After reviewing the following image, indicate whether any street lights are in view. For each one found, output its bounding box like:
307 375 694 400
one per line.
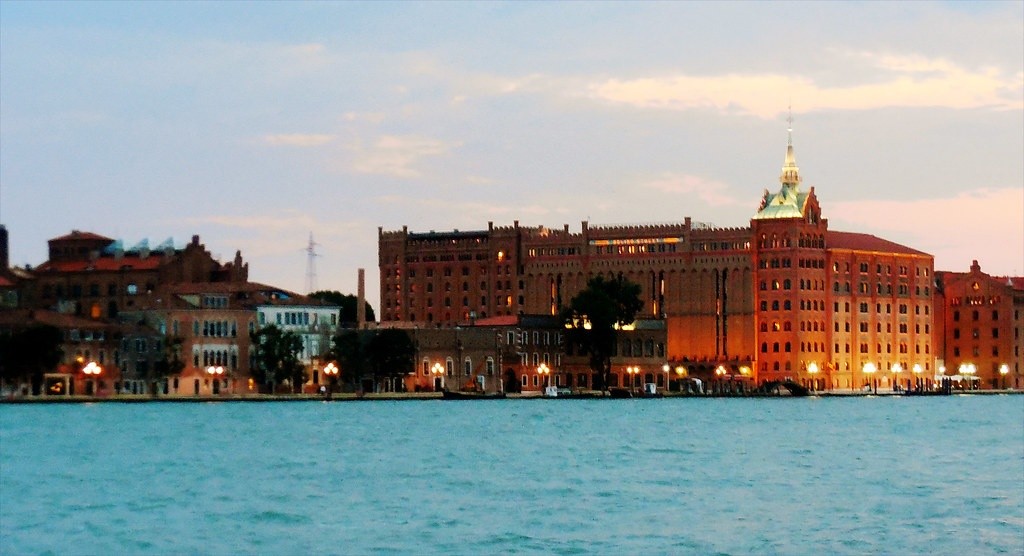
913 365 921 390
808 363 818 391
716 366 726 393
431 363 444 391
83 361 102 396
207 366 224 398
537 364 550 396
862 362 876 392
627 366 639 393
662 364 670 390
960 364 976 390
1000 365 1009 390
892 364 902 390
324 362 339 394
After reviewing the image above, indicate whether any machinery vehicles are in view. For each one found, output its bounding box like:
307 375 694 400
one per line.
462 355 488 392
